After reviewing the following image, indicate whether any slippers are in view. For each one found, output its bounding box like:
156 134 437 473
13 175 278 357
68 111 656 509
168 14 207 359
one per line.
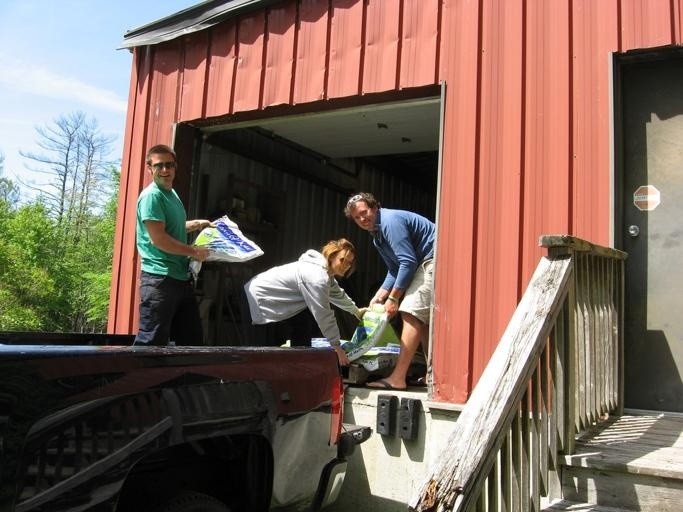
365 379 408 391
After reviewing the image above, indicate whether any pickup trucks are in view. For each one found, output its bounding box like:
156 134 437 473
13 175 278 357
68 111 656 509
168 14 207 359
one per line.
0 332 372 511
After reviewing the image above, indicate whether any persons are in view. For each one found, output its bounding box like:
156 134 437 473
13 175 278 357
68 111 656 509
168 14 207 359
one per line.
240 237 370 368
344 191 435 390
132 143 219 346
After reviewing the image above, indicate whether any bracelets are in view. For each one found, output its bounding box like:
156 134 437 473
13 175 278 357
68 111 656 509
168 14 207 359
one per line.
389 295 397 303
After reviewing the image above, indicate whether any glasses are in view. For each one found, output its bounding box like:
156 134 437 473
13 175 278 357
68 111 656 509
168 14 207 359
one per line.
152 161 176 169
